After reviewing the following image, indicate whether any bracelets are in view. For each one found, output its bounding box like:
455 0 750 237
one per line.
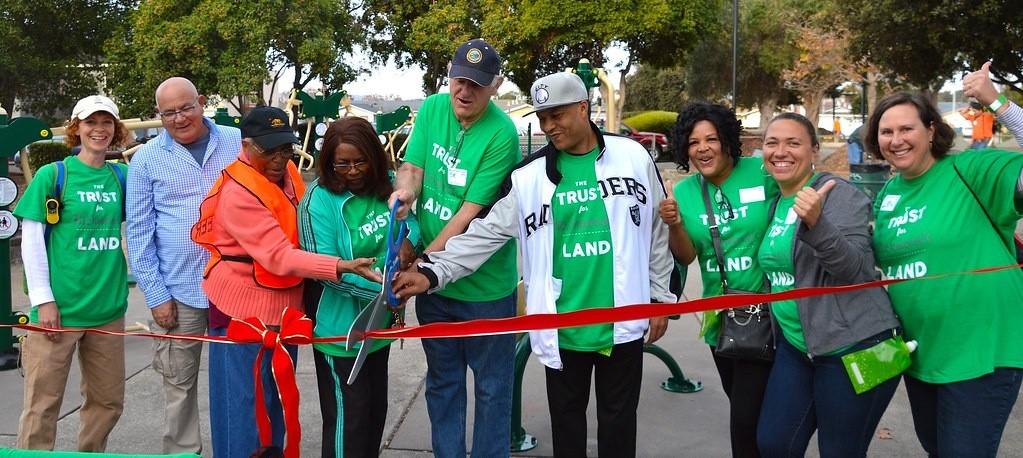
989 93 1008 112
419 251 434 265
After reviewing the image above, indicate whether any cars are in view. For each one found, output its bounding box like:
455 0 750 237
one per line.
532 118 670 163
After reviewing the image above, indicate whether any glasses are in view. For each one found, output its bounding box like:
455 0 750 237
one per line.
333 161 370 174
251 144 294 158
158 97 198 122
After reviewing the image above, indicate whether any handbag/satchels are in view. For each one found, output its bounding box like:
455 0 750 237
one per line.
715 287 775 364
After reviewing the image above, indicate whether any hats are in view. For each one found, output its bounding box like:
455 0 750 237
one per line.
71 95 120 123
240 106 300 150
520 72 588 118
449 38 500 87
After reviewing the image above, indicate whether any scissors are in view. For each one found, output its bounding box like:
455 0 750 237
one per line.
346 198 406 387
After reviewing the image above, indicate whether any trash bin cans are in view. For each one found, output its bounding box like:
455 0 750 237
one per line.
850 163 891 207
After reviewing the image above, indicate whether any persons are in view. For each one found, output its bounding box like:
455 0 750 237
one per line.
754 112 905 458
10 95 136 454
393 74 680 458
848 123 865 163
834 116 841 143
386 41 519 458
659 100 780 458
190 98 384 458
298 115 420 458
865 60 1023 458
124 76 244 458
961 101 996 150
